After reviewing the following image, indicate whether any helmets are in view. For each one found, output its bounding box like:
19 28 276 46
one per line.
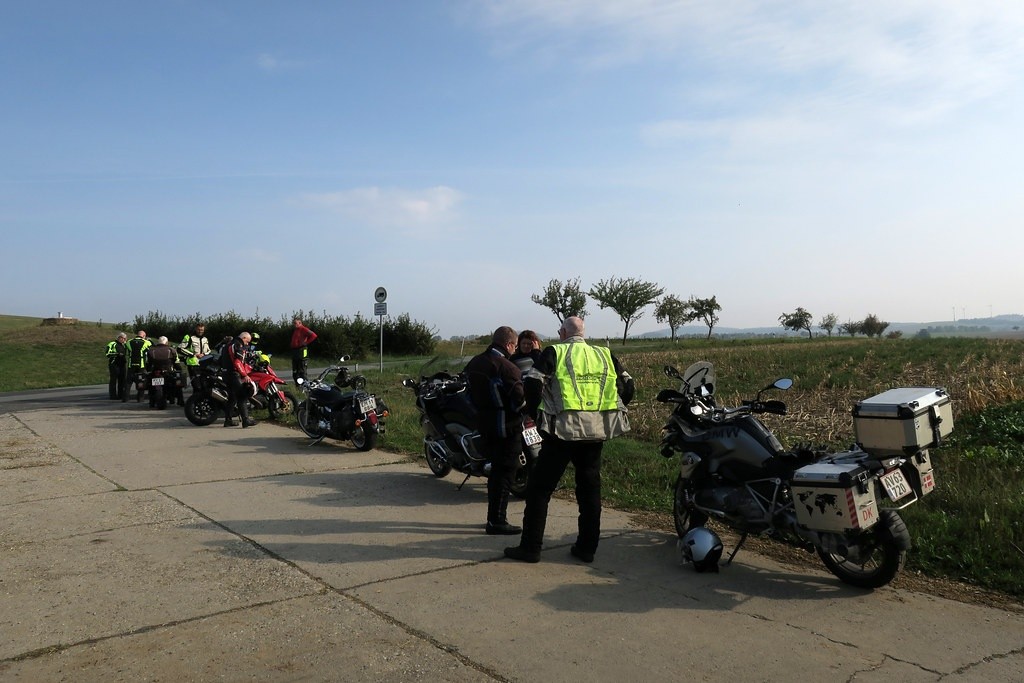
334 369 350 389
250 333 260 344
681 528 722 567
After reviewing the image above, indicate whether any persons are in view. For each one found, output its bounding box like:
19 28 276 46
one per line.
510 330 544 412
122 331 152 403
178 323 212 383
220 332 259 428
291 319 317 387
466 327 525 534
105 333 126 400
504 317 636 562
148 336 184 406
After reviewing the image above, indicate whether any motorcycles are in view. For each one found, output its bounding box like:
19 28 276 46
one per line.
401 355 546 499
296 354 390 452
656 360 955 590
134 354 183 410
183 352 299 426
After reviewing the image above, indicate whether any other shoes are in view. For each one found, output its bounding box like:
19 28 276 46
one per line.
571 545 595 564
176 399 186 406
485 517 525 535
242 419 259 428
138 399 146 403
503 545 539 563
224 420 239 427
